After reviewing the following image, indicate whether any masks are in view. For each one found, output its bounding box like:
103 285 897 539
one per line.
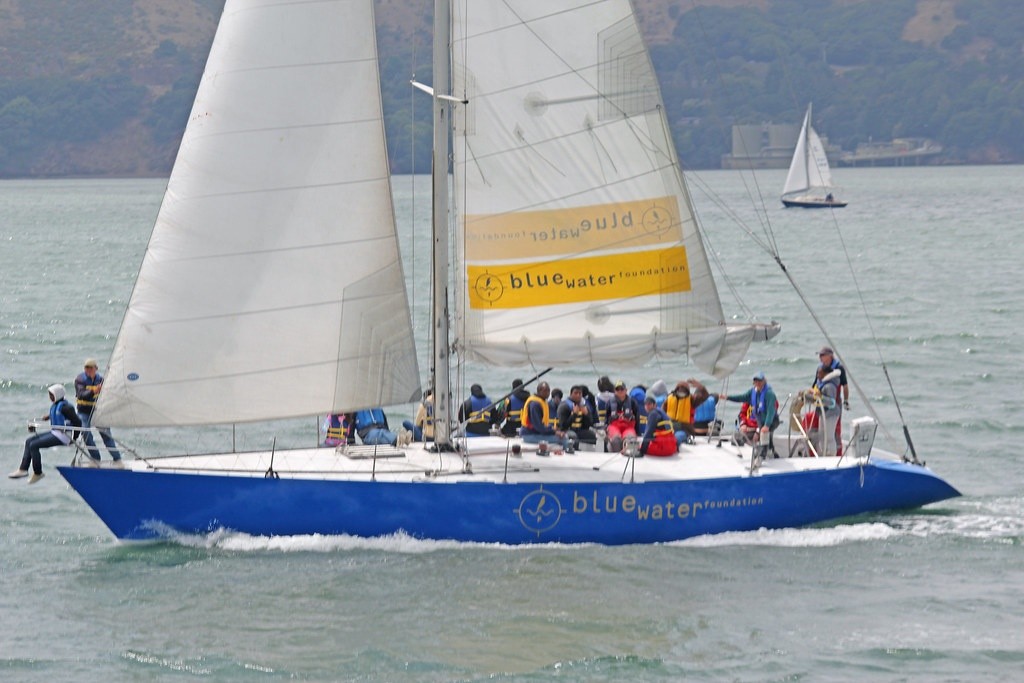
677 390 688 398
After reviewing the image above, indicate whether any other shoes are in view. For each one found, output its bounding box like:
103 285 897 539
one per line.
610 435 622 452
89 459 101 468
8 469 29 478
112 459 125 470
623 432 636 452
395 427 413 448
29 472 45 484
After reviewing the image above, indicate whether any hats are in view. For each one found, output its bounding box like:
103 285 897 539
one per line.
752 371 765 380
815 346 834 356
644 393 656 403
84 359 97 368
614 380 626 390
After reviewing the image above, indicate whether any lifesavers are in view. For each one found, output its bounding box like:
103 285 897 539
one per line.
790 396 806 431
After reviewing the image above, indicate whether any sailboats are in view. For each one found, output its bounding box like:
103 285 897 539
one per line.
55 0 963 545
781 102 849 207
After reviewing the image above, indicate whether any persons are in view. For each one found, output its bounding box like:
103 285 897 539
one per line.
548 389 563 428
809 347 849 455
519 381 570 450
495 379 530 437
403 389 434 442
579 386 600 424
7 383 82 483
355 407 413 449
595 376 724 457
557 384 608 452
320 412 356 448
458 384 497 436
75 357 124 469
719 371 780 459
